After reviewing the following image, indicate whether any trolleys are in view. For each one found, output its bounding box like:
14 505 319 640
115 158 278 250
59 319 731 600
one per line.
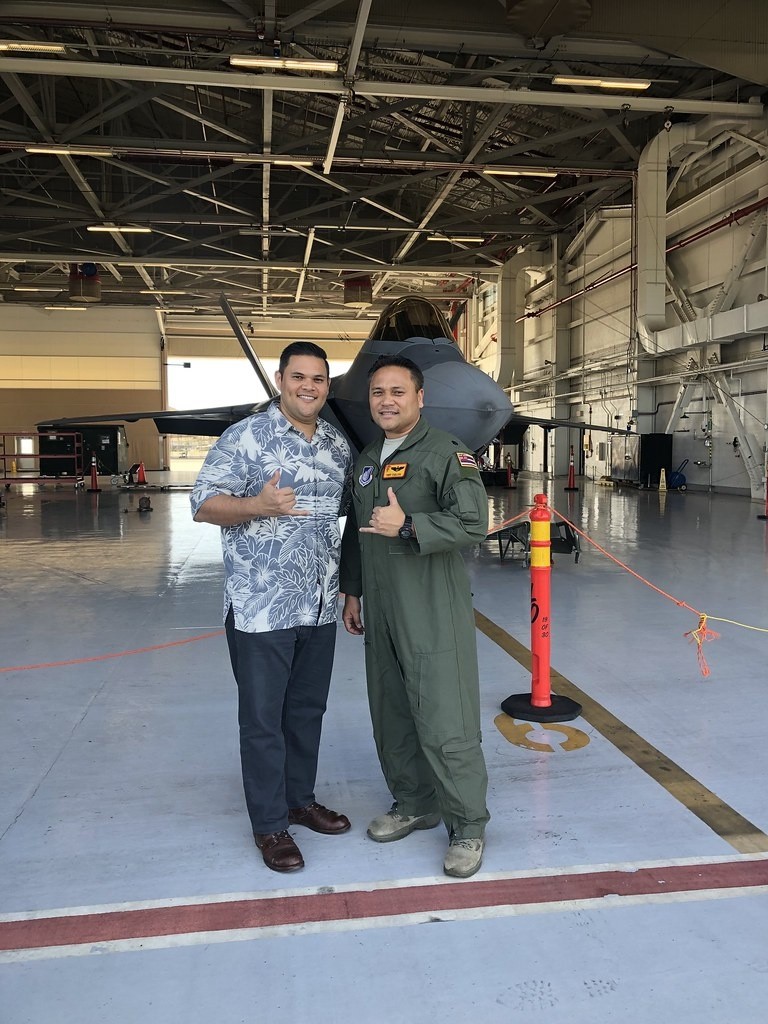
669 458 690 492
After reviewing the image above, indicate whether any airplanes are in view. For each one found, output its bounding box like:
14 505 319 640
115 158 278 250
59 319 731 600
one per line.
34 293 644 466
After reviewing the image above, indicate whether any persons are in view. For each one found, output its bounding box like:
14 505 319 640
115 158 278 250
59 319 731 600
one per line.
504 452 512 464
188 342 354 873
339 355 489 878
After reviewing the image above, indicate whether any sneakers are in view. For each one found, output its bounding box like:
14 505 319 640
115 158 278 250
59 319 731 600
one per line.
366 810 442 843
444 838 485 878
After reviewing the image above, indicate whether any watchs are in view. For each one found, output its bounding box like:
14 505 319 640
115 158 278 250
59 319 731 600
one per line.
398 516 413 540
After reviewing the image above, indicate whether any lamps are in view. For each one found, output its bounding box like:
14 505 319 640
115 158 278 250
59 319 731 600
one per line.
551 73 651 89
87 222 151 232
239 225 300 236
25 144 114 157
229 55 338 70
233 157 313 166
138 289 186 294
13 285 63 292
427 232 484 242
483 166 557 177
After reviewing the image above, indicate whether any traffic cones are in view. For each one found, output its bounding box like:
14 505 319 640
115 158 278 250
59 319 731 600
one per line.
658 468 668 492
500 453 519 490
563 446 578 491
86 450 104 494
11 461 18 473
135 461 148 485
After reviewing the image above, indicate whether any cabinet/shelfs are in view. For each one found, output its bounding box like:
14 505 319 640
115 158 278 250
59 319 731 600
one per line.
610 432 673 482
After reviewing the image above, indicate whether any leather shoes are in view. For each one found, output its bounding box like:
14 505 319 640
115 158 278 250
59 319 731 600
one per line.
288 801 350 835
254 830 305 872
